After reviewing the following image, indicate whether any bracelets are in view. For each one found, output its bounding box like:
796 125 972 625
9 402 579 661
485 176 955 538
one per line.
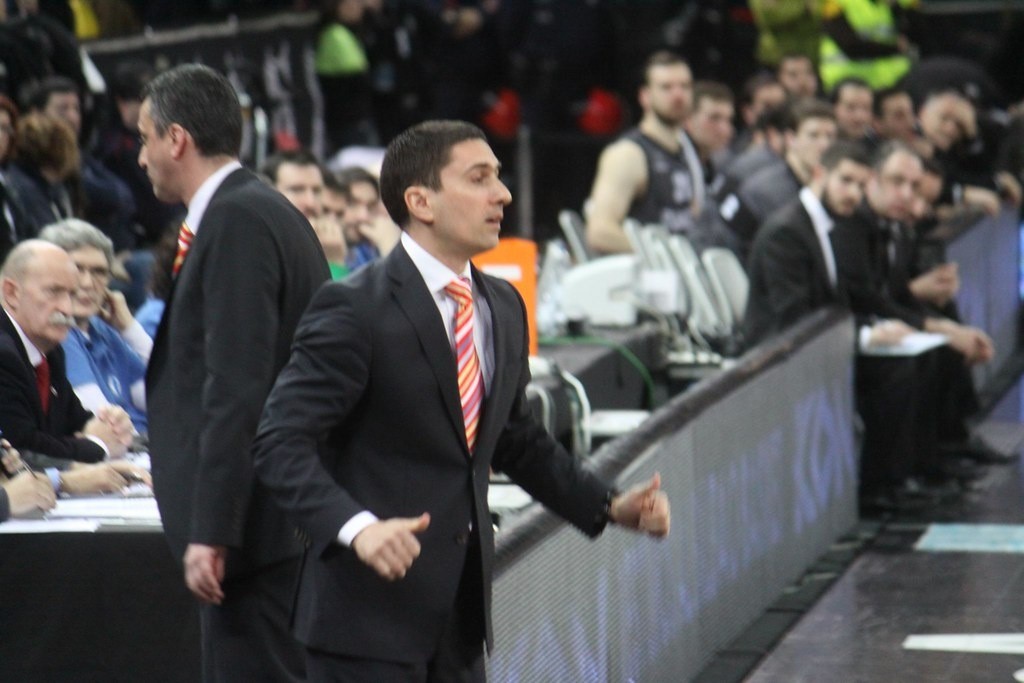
44 467 69 498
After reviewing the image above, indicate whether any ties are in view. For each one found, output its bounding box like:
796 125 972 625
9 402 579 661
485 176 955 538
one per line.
442 277 486 458
173 220 193 281
32 355 51 415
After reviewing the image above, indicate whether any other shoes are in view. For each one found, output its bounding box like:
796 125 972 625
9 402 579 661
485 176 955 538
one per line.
859 432 1005 523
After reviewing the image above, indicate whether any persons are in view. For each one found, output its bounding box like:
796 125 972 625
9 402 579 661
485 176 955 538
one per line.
248 121 668 683
135 61 333 683
0 0 393 526
314 0 1024 515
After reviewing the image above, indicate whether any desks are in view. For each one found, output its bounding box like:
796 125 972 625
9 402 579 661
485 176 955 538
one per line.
0 320 667 683
